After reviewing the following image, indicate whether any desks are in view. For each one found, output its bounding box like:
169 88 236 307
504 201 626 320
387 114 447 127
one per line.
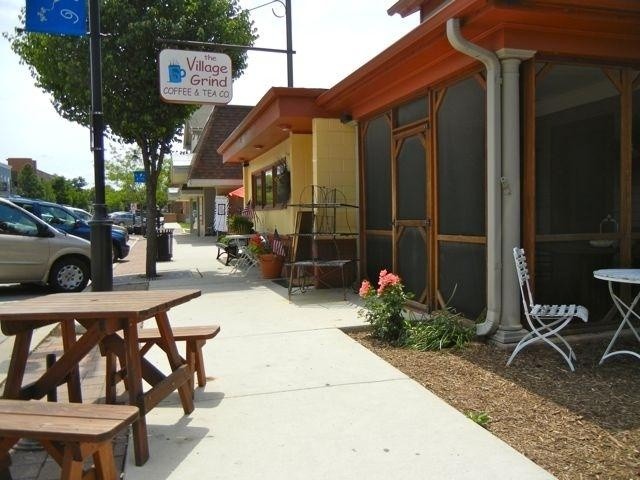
593 263 640 369
0 292 199 468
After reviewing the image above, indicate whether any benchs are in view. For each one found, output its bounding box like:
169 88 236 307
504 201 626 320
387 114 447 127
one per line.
216 239 239 267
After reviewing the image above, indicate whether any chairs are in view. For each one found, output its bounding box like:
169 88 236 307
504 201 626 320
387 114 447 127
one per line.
504 245 589 372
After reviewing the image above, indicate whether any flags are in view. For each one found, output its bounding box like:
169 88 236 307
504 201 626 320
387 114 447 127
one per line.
242 201 256 219
272 229 289 257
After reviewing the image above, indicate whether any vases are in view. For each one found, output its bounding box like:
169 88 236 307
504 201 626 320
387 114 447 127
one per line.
259 253 283 279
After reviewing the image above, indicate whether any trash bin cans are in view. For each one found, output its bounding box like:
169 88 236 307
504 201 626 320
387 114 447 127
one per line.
157 229 173 262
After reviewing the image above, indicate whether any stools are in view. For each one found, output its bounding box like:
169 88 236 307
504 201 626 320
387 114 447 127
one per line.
0 396 140 475
114 326 223 399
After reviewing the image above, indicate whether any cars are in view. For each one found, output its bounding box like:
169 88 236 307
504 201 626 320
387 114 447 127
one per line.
0 198 91 292
10 199 146 258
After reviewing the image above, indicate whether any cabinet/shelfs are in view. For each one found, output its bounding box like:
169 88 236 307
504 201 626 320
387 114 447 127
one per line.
281 183 363 302
